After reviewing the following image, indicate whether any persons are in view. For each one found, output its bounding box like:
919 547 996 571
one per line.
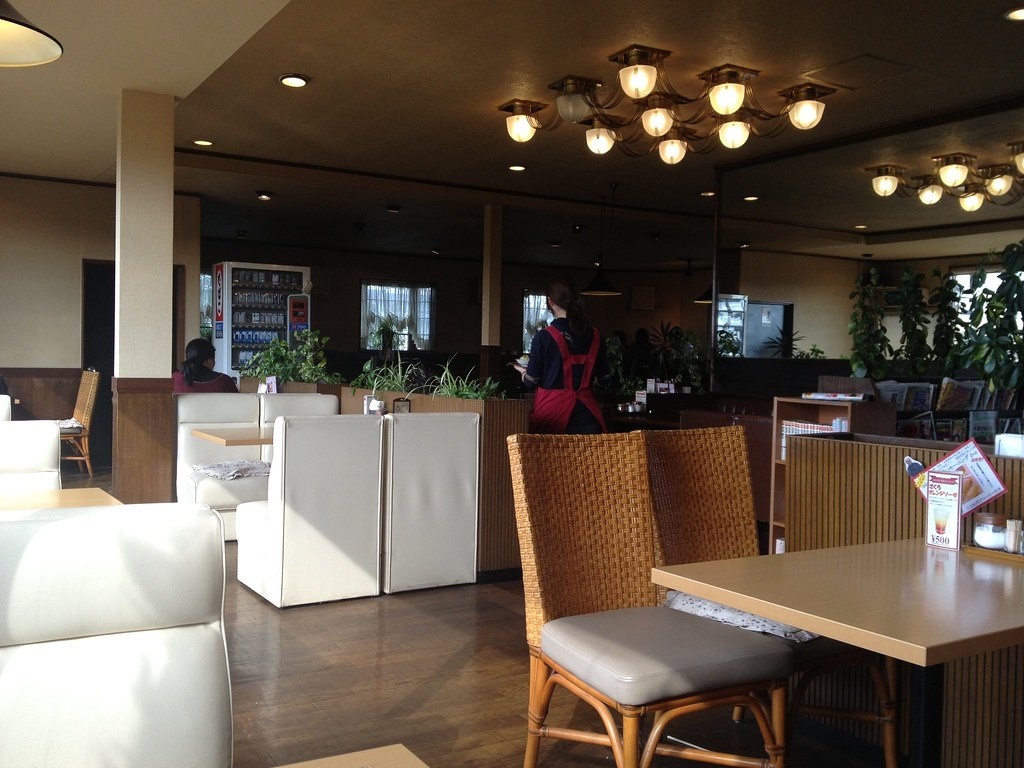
667 326 698 357
514 278 607 435
614 328 656 363
173 338 238 392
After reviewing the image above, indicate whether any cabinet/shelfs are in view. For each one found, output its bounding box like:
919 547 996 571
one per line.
767 394 897 557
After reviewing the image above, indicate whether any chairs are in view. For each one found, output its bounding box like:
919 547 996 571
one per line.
506 432 795 768
642 426 901 768
58 370 100 478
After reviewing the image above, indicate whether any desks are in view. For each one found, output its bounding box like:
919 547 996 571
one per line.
651 536 1024 768
0 487 125 512
191 428 274 446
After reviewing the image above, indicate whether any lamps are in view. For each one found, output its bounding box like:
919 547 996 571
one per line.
580 196 623 296
497 44 836 166
865 140 1024 211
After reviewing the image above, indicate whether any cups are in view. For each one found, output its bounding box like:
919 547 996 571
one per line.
934 507 949 534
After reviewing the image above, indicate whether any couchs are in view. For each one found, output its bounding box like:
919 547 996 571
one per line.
0 502 234 768
0 420 62 491
175 391 480 609
679 407 773 524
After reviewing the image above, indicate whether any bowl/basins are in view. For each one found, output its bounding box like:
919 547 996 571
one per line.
516 359 529 367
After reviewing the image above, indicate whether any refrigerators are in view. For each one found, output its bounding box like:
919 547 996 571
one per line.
212 261 310 394
